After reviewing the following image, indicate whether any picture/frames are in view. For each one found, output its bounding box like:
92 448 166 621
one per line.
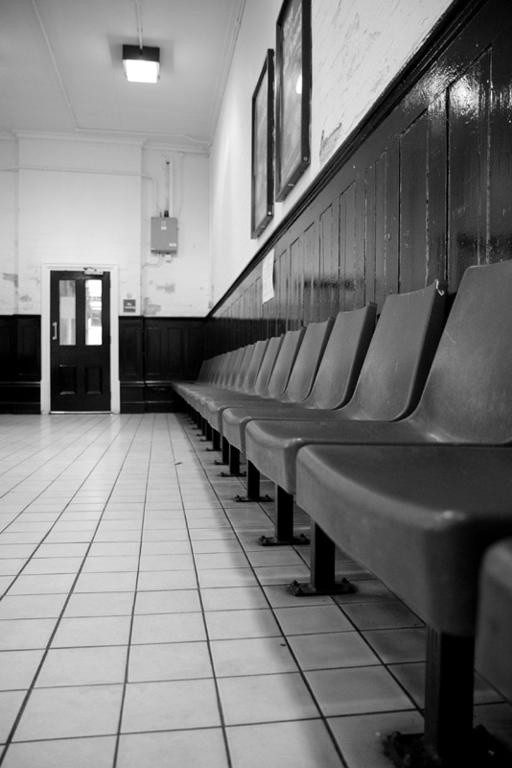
250 0 309 239
